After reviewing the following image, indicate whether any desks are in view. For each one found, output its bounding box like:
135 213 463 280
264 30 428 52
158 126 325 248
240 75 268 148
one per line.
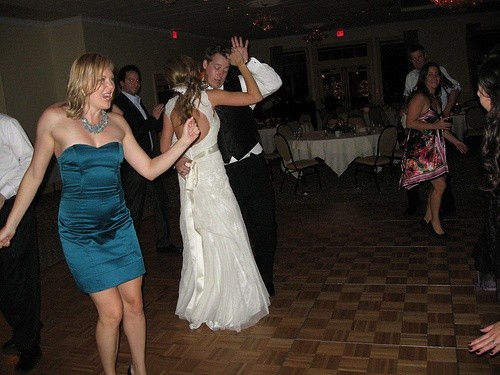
254 119 287 154
452 108 472 142
281 127 400 178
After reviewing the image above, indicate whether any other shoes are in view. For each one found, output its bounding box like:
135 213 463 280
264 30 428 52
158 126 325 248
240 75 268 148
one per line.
404 200 423 217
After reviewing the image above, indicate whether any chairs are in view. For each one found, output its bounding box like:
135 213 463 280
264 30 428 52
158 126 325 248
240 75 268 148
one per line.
464 107 488 145
264 107 402 199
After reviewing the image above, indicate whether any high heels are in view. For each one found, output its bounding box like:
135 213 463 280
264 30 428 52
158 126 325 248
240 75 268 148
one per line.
421 218 429 230
428 221 447 238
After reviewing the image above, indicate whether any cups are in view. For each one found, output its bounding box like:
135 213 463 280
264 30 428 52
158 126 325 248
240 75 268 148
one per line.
353 126 357 135
444 118 453 129
324 130 328 136
368 121 382 135
335 131 342 138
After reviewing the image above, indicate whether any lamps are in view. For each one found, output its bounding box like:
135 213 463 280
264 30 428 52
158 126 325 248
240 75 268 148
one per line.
250 4 284 33
302 16 328 47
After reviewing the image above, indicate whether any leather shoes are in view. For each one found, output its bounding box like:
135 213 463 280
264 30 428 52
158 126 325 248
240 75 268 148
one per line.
2 341 16 351
157 243 182 253
14 349 42 373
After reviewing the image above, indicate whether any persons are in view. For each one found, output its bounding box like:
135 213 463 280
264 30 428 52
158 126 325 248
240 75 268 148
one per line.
115 65 178 254
403 43 462 191
0 113 43 375
470 44 500 304
398 61 469 238
358 97 389 127
0 54 200 375
161 47 270 332
468 321 500 356
202 36 282 292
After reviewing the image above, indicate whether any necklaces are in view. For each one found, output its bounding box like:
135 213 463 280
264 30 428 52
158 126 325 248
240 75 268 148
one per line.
79 110 108 133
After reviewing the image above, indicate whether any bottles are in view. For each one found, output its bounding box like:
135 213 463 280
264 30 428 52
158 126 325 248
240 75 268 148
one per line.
296 127 302 140
